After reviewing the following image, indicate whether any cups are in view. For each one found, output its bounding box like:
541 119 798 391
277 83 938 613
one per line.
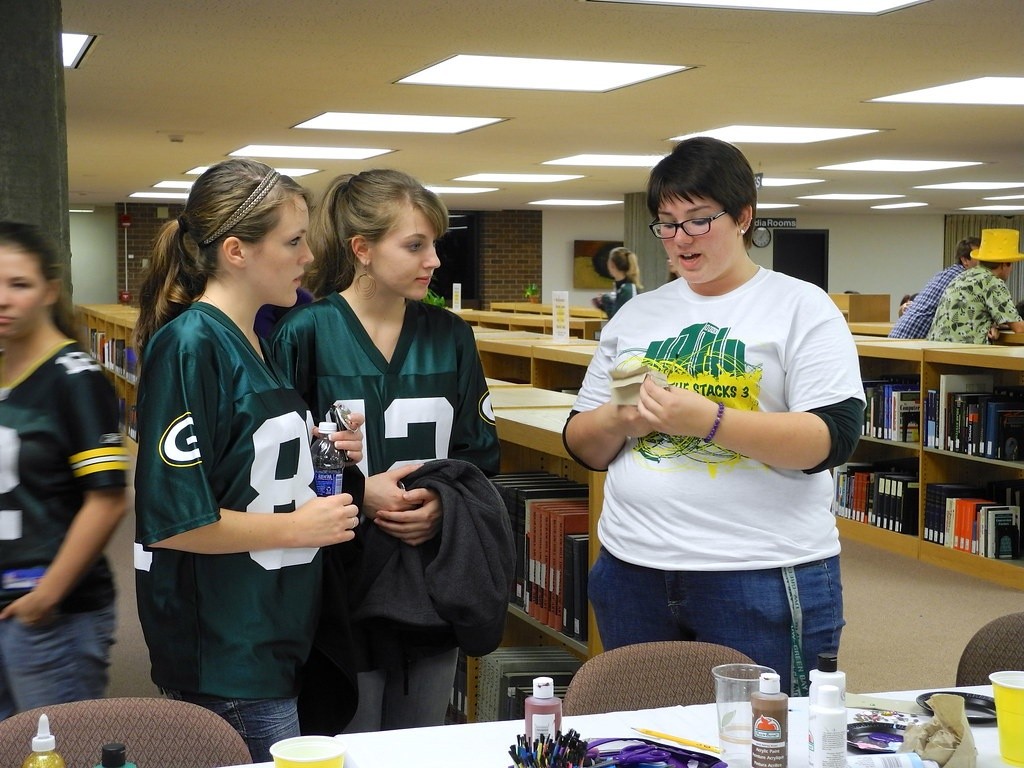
269 735 347 768
988 670 1024 768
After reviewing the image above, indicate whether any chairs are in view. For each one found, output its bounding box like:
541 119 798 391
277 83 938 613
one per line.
956 612 1024 688
561 641 762 716
0 698 254 768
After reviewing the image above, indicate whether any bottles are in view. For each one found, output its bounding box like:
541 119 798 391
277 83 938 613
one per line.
807 653 849 768
94 743 137 768
310 422 347 497
711 663 777 745
749 674 788 767
22 714 67 768
525 677 562 750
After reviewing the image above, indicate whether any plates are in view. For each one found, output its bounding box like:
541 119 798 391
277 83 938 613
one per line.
846 722 907 753
915 691 997 724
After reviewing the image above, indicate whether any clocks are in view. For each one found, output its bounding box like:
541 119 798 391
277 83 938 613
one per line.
752 226 771 248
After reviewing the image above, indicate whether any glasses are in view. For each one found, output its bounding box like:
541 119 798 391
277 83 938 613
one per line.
649 208 728 239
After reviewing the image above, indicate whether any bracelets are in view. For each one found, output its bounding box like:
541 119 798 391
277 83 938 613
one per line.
703 402 725 444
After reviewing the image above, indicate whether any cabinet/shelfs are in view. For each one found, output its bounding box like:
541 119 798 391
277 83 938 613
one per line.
73 294 1024 727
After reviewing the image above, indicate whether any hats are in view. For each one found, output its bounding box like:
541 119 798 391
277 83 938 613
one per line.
970 229 1024 263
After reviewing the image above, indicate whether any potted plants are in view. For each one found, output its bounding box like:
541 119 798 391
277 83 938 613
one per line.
526 284 539 303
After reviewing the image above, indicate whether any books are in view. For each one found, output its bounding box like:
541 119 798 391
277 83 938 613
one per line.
446 645 584 722
487 472 591 642
831 374 1024 559
82 327 141 443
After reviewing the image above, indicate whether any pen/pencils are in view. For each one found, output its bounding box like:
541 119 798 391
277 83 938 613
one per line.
630 726 726 755
510 727 589 768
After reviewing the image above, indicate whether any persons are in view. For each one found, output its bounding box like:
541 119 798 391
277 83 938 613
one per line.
0 220 132 720
562 137 867 697
926 228 1024 345
592 247 641 317
268 169 500 735
130 159 366 764
887 236 981 339
666 257 681 281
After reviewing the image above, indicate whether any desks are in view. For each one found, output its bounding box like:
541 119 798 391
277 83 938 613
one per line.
332 686 1024 768
